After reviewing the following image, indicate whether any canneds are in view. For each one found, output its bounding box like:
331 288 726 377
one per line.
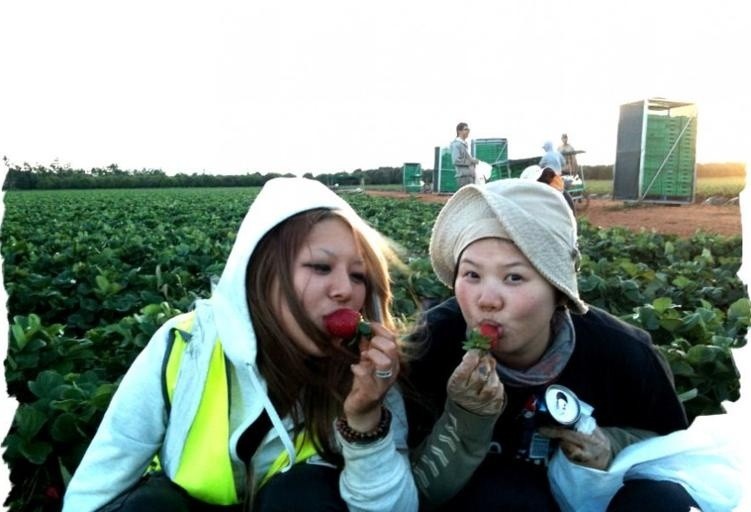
513 383 581 467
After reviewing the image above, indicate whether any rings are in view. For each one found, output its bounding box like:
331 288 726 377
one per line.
374 367 394 378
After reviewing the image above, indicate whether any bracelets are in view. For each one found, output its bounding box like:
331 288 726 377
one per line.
335 403 392 444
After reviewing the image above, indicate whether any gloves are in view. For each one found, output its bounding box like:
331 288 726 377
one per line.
446 348 505 416
540 426 611 469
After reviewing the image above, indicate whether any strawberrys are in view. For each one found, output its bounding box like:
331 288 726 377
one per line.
322 308 364 337
474 322 499 350
44 484 62 508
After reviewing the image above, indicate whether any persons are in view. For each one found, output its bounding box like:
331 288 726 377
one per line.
396 175 700 512
538 167 556 185
539 140 566 178
59 175 420 512
449 122 493 187
557 132 578 173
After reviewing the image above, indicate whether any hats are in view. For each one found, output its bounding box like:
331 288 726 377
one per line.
427 178 588 316
